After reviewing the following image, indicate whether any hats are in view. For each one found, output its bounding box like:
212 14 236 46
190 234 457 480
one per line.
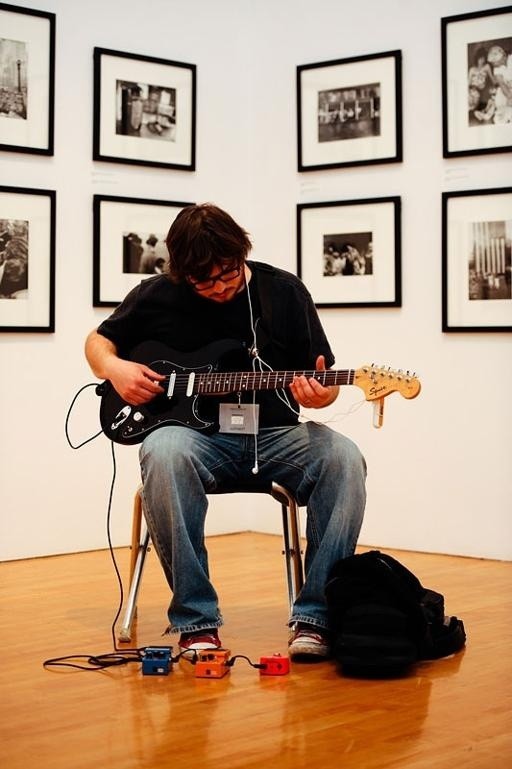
146 234 157 244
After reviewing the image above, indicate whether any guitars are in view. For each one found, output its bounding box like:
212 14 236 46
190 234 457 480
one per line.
99 361 421 444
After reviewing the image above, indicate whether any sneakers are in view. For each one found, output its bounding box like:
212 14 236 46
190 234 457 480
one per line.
288 620 330 659
178 628 222 661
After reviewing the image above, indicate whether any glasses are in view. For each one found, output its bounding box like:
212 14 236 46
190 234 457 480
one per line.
187 259 241 291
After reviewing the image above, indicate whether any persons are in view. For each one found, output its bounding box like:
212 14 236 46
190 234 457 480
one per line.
121 232 169 275
85 203 369 663
321 240 372 277
468 45 512 126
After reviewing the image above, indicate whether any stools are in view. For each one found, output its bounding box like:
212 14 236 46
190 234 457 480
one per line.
120 473 304 649
